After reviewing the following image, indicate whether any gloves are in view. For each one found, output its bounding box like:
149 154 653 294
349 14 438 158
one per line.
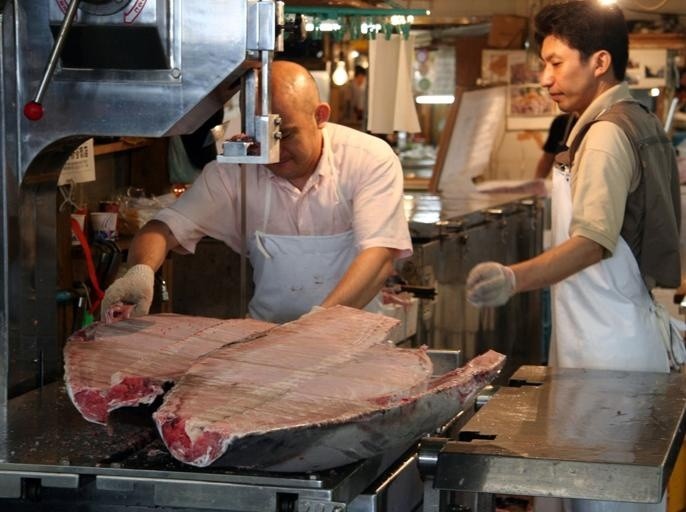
462 258 518 313
99 261 156 326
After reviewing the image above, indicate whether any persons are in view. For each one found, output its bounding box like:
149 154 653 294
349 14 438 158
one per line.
465 0 681 512
101 59 415 328
340 65 366 121
536 113 578 179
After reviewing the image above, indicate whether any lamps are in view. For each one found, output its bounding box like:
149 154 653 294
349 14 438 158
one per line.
331 31 349 86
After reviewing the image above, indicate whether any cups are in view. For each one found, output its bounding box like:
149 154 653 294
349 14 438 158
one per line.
90 210 118 243
70 213 86 246
